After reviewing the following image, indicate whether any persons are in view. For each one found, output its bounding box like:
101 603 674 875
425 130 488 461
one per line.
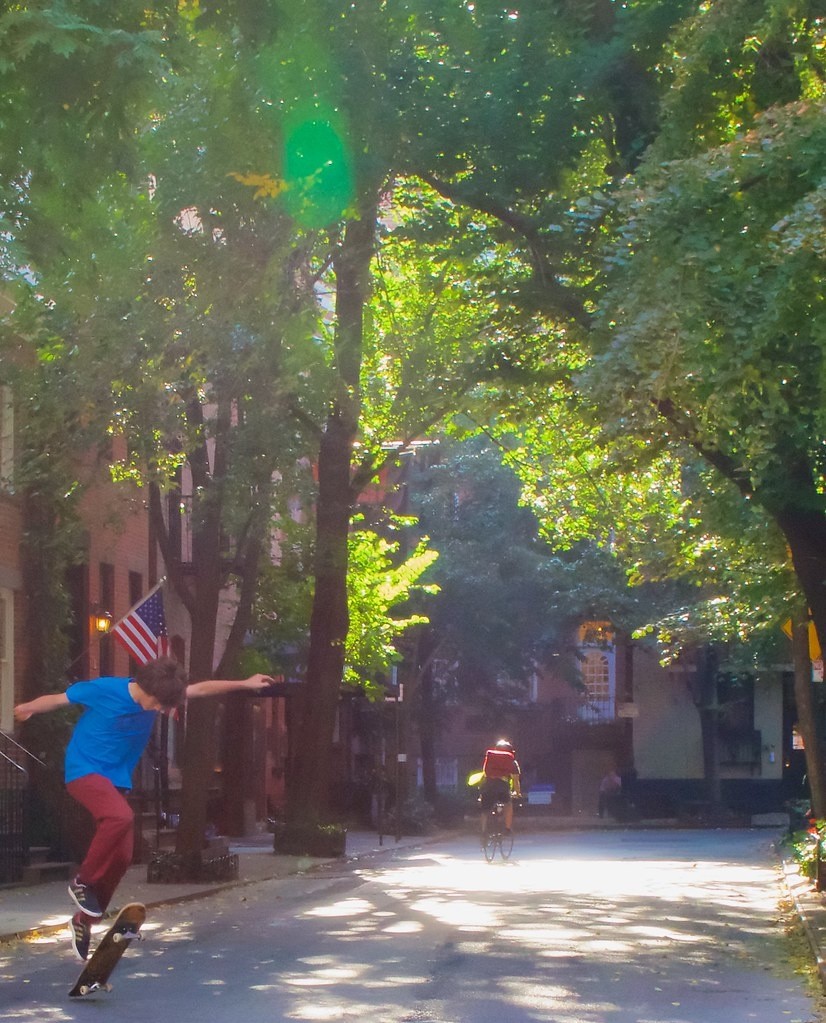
10 674 273 962
597 768 621 817
480 739 521 849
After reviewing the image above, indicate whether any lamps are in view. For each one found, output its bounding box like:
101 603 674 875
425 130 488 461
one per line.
91 602 113 631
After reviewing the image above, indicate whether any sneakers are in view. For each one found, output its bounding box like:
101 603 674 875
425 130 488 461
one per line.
68 876 103 917
69 913 91 962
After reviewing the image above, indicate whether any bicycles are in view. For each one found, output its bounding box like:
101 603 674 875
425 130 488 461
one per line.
477 787 521 863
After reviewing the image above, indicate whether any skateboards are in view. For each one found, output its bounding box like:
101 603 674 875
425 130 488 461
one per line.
66 901 148 998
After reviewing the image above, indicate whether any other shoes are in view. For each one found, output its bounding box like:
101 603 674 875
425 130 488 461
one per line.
504 830 513 838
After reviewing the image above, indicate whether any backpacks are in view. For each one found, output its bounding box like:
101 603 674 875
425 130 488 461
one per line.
484 749 515 779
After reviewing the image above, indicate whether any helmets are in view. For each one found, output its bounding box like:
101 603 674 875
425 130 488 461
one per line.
496 741 512 752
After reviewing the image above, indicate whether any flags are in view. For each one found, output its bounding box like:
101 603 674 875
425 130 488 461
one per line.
111 587 179 721
